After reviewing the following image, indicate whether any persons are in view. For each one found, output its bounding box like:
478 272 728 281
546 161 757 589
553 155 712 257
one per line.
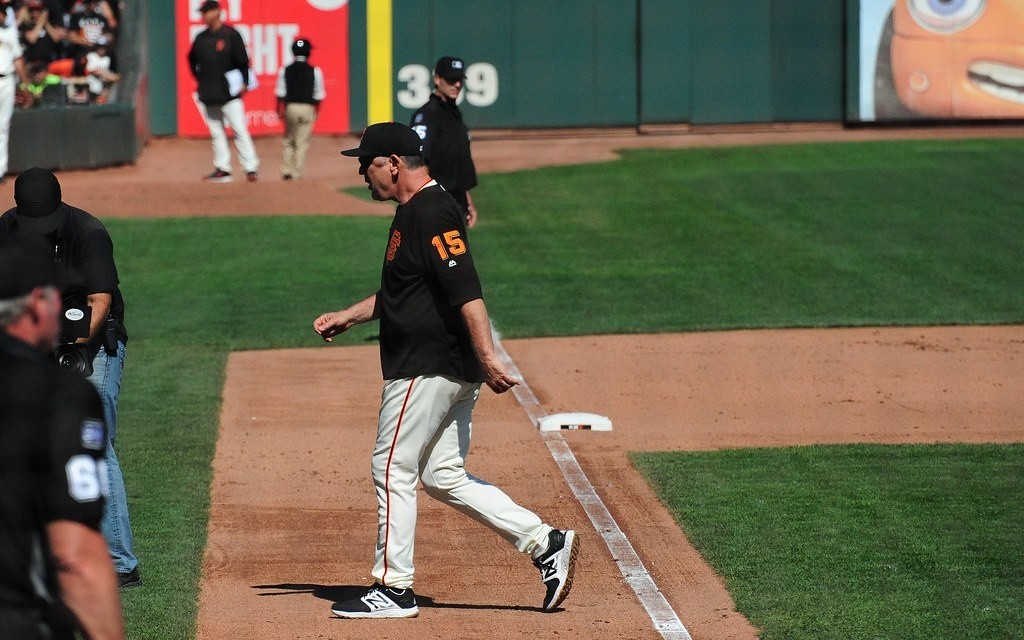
189 0 260 183
0 169 145 640
273 39 326 181
409 56 478 230
0 0 121 184
313 120 583 620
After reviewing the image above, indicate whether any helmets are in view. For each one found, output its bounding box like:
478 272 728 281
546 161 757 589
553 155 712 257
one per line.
292 40 310 57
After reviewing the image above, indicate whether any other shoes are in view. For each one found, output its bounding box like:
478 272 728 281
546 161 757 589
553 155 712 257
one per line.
283 174 292 180
116 566 142 589
201 167 233 183
247 172 257 182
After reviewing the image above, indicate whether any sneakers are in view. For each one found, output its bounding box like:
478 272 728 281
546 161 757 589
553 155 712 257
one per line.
331 582 419 619
532 529 580 612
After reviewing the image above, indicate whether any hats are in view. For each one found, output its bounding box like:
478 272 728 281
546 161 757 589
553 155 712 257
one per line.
198 0 219 14
436 56 467 83
0 215 84 290
340 120 423 158
14 167 62 234
